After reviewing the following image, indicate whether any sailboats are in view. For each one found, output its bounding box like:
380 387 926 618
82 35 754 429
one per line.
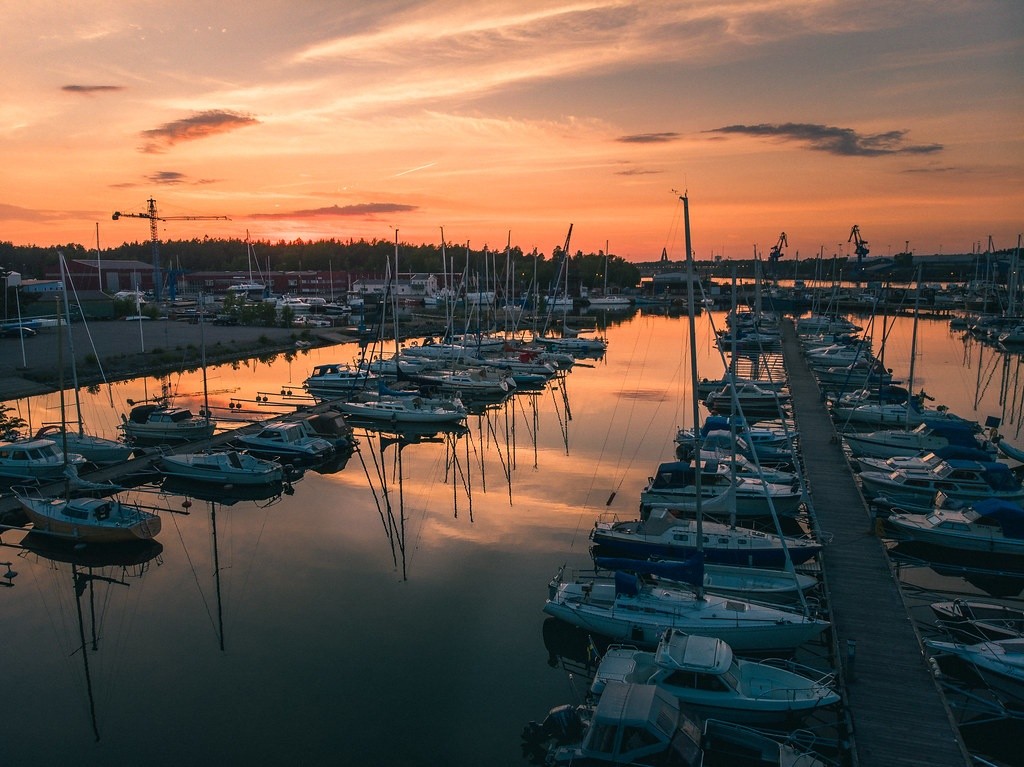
0 190 1024 766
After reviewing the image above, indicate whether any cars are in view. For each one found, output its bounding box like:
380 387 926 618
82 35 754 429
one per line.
0 326 37 339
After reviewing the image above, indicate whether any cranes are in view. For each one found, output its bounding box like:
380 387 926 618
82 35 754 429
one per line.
110 197 233 303
767 231 788 288
847 225 870 287
